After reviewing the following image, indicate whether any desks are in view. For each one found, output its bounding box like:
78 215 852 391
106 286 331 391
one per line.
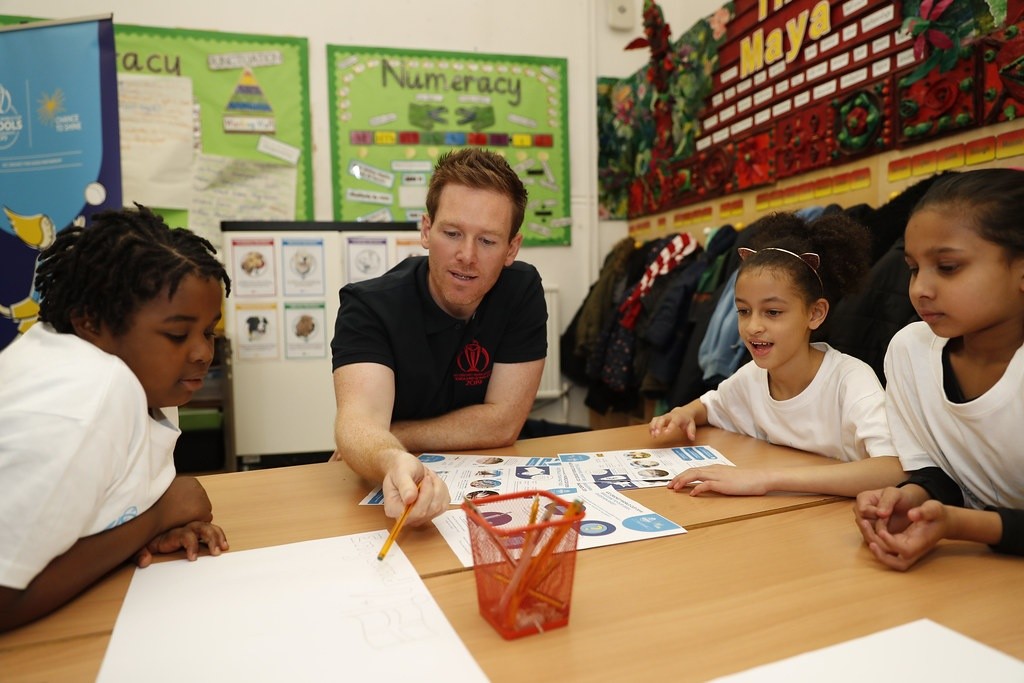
177 328 238 472
0 423 1024 683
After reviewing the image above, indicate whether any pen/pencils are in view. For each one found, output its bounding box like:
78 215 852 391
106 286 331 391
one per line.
375 475 430 562
462 491 587 631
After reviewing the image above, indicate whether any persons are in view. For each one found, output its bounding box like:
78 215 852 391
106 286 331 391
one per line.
0 200 232 630
649 212 910 497
853 168 1023 569
330 149 549 527
